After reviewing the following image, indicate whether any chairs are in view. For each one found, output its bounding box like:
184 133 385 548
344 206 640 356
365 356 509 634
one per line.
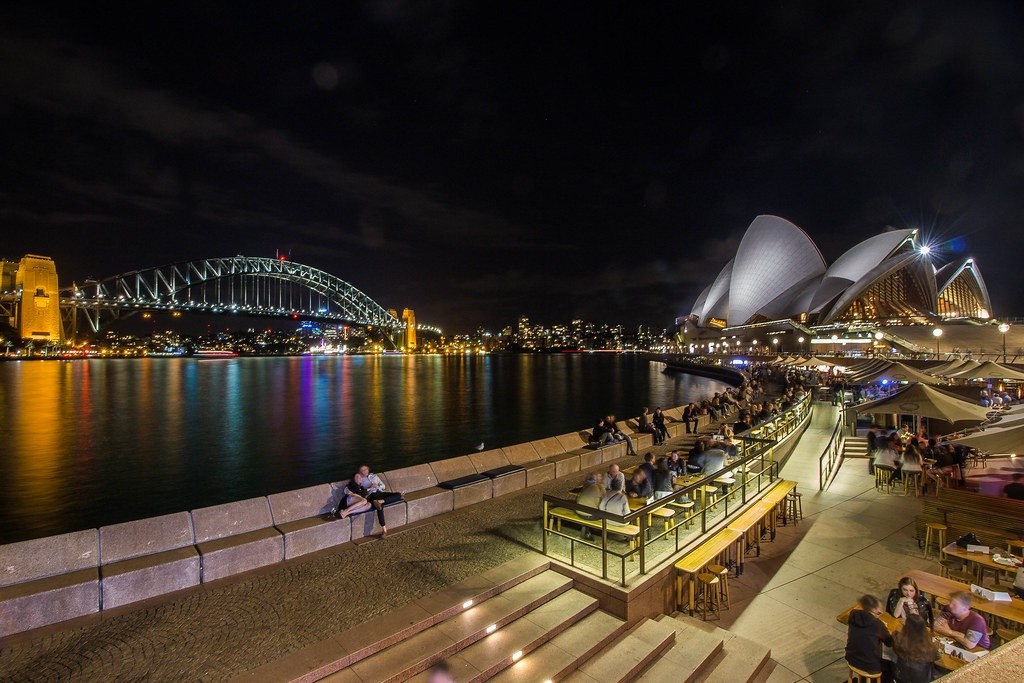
965 447 978 467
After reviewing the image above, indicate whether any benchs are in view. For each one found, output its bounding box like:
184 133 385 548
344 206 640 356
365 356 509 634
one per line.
674 528 744 618
762 480 798 537
0 394 811 639
727 499 777 556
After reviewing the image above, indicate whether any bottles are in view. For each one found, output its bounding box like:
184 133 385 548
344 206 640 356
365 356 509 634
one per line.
907 596 916 614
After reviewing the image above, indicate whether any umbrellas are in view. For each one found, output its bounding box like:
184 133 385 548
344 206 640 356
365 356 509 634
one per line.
767 356 1024 455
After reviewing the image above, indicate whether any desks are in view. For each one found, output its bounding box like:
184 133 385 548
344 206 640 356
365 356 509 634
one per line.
834 602 988 670
864 437 909 459
623 466 703 516
570 486 648 550
897 570 1024 630
942 542 1023 583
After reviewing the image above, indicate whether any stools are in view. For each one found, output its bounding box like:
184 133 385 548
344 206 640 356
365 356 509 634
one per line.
922 522 948 561
845 661 883 683
784 496 798 526
874 466 959 496
788 491 802 520
697 573 722 621
707 565 729 610
927 559 1022 645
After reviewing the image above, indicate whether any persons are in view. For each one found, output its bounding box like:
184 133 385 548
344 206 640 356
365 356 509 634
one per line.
336 473 373 518
844 380 1024 683
344 464 402 535
575 358 848 541
710 347 1024 358
